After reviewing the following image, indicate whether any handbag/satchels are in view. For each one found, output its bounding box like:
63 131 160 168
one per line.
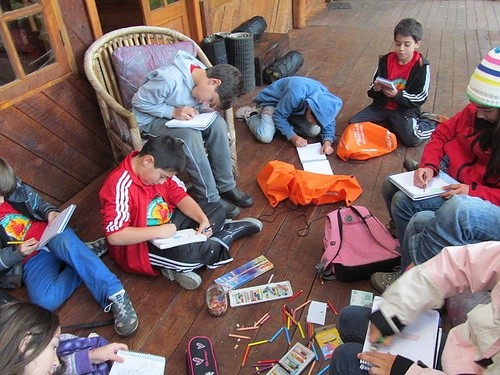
337 121 398 163
256 160 363 207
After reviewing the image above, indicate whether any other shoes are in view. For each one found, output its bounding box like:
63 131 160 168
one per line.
214 186 254 217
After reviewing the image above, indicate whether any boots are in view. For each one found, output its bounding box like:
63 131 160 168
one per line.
211 217 263 252
160 266 204 290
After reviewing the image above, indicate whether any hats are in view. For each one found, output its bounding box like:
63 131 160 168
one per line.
466 45 500 108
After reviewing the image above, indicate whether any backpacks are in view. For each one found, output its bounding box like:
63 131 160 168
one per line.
317 205 404 282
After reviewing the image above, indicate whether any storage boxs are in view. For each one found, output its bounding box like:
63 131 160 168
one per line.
252 31 291 86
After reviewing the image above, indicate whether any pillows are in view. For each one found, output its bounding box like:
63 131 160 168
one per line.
112 41 198 110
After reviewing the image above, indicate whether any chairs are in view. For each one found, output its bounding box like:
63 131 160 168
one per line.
83 25 239 196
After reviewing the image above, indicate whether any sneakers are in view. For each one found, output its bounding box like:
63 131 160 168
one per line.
235 104 255 122
108 288 139 337
421 110 450 124
84 236 108 257
369 268 402 294
402 158 421 171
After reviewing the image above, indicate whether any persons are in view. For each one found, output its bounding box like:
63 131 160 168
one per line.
327 239 500 375
98 134 264 290
129 49 255 218
379 45 500 262
235 75 344 156
0 156 139 336
369 193 500 325
8 14 55 83
348 16 452 148
1 300 129 375
